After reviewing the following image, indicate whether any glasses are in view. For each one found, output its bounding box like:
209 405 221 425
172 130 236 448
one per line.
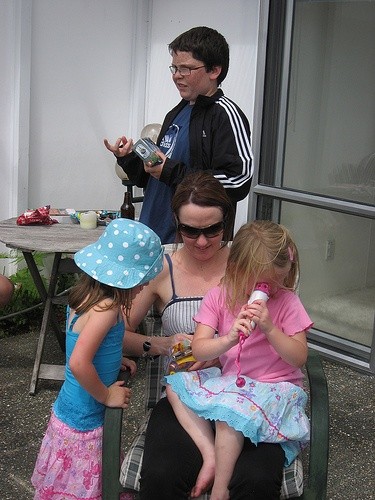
178 220 224 238
169 62 215 76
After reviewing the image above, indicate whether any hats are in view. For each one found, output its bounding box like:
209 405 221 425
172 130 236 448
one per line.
74 218 166 288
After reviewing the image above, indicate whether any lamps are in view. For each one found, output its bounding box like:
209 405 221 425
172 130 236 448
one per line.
115 123 163 202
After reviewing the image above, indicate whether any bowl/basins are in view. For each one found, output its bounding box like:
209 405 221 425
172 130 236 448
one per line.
50 215 78 225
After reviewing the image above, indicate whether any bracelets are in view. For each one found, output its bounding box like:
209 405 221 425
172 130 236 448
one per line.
143 336 154 356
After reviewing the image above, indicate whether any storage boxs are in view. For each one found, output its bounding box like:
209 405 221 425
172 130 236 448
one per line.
131 137 162 166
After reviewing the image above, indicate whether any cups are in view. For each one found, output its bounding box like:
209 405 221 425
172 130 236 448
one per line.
80 214 100 230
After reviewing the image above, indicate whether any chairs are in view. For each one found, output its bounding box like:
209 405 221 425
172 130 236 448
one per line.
100 242 330 500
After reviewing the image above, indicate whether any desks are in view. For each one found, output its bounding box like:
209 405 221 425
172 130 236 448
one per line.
0 208 123 396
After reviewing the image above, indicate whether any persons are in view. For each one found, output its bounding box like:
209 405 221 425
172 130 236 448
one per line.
103 26 254 241
162 218 314 500
29 219 165 500
122 170 286 500
